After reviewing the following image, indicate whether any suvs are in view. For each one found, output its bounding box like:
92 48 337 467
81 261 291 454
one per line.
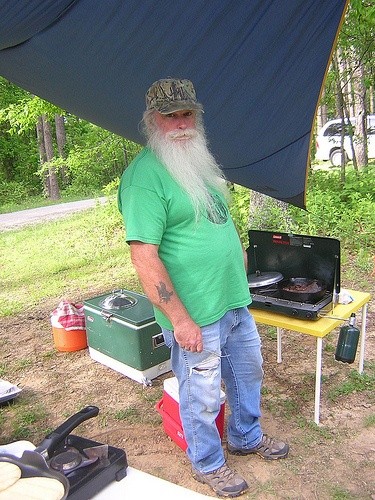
314 115 375 167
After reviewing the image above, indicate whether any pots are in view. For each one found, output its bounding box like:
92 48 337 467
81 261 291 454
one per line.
258 278 327 304
0 406 99 500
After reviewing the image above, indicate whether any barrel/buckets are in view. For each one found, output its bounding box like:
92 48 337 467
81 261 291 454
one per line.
50 303 87 352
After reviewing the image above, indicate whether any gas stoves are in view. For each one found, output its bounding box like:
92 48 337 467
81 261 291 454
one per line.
44 435 127 500
245 230 340 321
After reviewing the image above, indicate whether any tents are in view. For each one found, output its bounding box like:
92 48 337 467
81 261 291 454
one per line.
0 0 375 249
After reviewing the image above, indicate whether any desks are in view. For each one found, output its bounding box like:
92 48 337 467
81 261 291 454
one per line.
248 287 372 426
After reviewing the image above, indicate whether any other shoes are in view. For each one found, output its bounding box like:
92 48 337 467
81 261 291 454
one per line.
190 461 249 497
226 433 291 460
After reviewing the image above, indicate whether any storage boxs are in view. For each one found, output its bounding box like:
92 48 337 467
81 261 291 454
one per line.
83 287 172 383
155 376 227 455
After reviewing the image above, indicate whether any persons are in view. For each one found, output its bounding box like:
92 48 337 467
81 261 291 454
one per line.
118 78 290 498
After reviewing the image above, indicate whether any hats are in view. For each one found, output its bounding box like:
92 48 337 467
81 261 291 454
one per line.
145 77 205 115
